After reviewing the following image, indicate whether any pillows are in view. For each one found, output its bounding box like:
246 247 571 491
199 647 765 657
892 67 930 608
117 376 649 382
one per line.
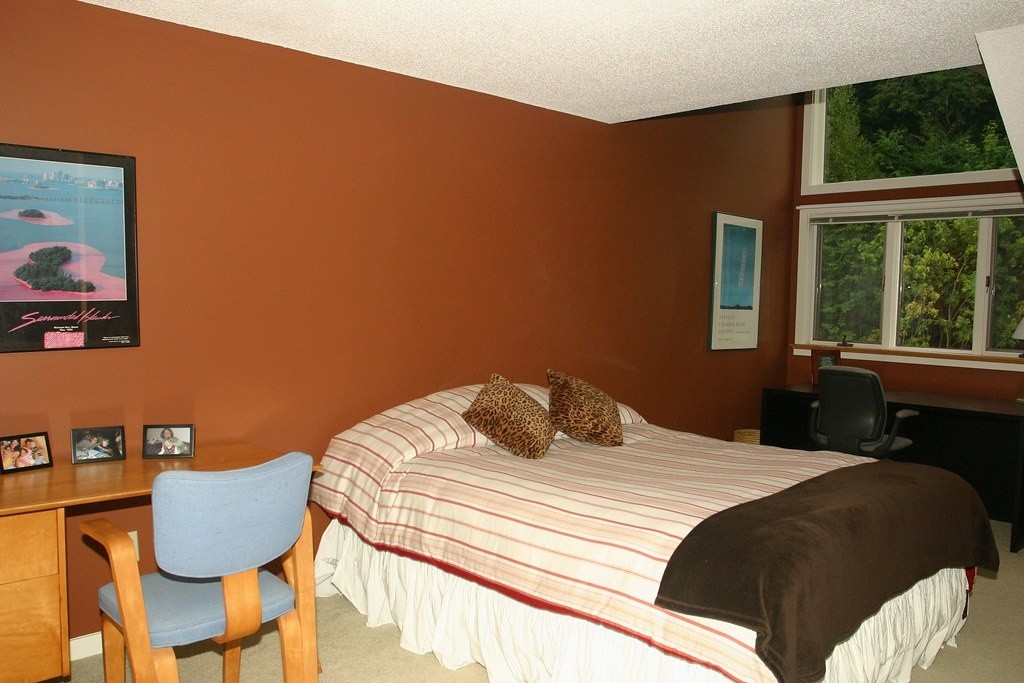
545 369 623 446
461 373 558 458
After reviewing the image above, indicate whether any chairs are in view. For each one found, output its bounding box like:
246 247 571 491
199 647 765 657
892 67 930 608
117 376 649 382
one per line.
809 365 920 458
78 451 321 683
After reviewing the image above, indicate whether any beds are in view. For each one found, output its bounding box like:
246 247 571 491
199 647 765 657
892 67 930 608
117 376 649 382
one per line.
308 382 1000 683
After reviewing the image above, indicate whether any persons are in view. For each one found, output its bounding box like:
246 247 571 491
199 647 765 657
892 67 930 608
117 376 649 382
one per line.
158 428 187 454
0 439 44 470
76 430 121 456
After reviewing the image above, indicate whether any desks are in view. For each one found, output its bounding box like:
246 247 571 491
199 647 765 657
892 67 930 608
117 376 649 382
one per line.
761 386 1024 552
0 440 325 683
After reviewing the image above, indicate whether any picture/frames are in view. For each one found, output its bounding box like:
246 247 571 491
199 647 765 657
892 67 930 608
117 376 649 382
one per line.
709 211 764 350
142 423 195 458
0 432 53 475
71 426 126 465
811 348 841 384
